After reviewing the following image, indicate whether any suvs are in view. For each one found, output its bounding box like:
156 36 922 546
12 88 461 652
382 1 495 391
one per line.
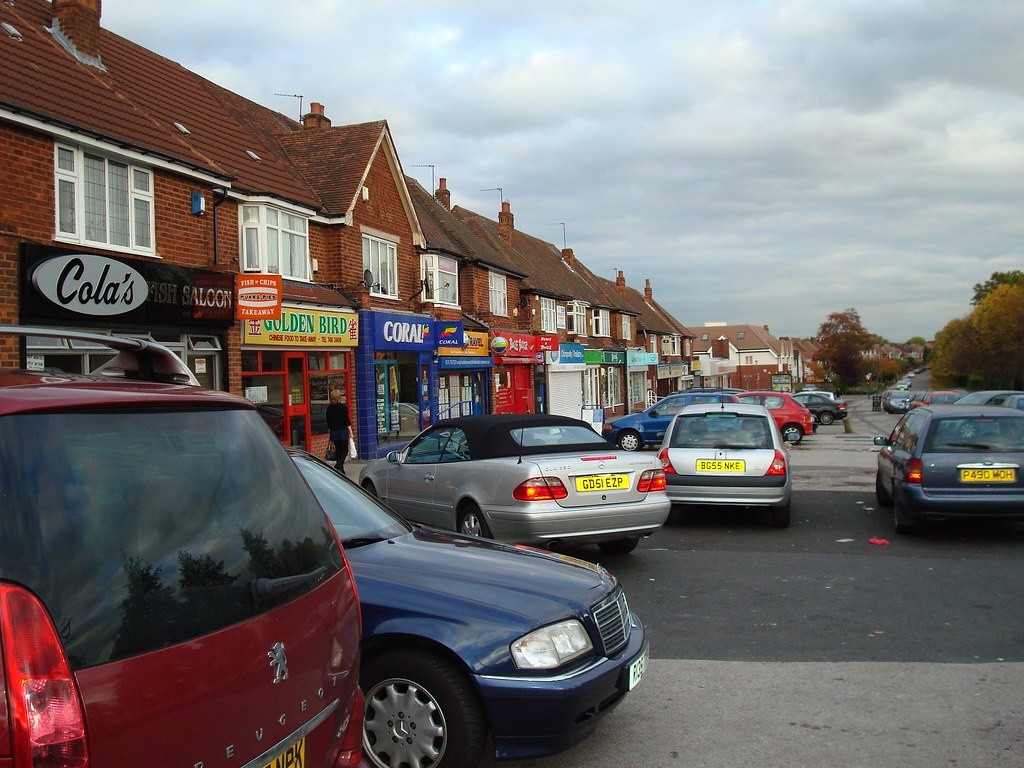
678 386 747 394
0 319 368 768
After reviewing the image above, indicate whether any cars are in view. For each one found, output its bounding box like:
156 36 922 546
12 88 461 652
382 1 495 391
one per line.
656 403 793 530
310 400 330 435
884 367 961 414
357 413 673 551
601 394 742 451
399 403 420 417
792 391 848 425
257 405 283 437
735 392 813 447
282 442 650 768
873 405 1024 533
952 390 1024 409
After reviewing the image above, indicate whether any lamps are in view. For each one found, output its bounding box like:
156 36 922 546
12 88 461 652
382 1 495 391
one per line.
516 296 528 309
408 277 430 300
358 269 374 287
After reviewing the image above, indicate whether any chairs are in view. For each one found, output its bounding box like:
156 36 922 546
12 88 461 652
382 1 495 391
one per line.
742 419 762 439
935 426 961 444
689 420 709 435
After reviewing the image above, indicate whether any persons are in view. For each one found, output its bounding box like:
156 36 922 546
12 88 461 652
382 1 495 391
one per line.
326 390 354 474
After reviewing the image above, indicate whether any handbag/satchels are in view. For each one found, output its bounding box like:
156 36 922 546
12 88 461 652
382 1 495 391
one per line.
324 436 338 461
349 437 359 461
